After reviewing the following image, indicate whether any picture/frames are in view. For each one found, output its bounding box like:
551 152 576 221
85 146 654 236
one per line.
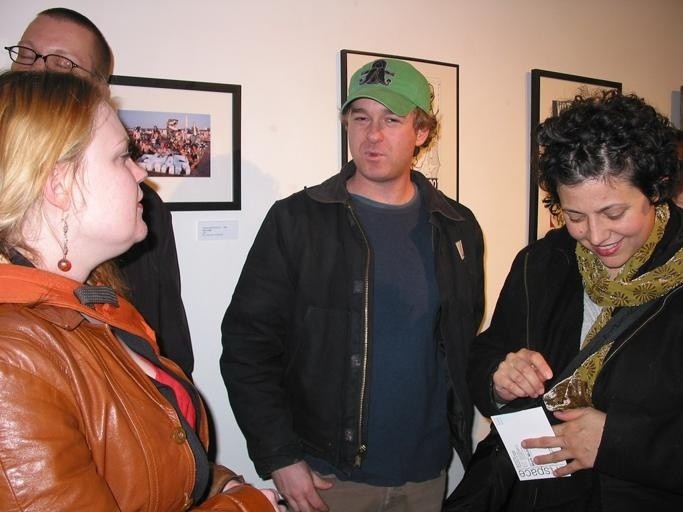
341 48 459 200
107 75 241 211
529 68 622 244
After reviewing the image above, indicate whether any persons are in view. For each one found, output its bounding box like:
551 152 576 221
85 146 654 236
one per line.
2 8 195 385
219 58 486 511
441 90 682 511
1 71 286 510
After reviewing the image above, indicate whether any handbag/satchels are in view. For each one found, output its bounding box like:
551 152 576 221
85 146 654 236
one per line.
443 398 555 512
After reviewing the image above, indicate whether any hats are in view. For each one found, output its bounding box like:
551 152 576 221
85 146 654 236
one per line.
341 59 432 116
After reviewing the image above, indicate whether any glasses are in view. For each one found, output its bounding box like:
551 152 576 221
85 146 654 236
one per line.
4 46 94 78
